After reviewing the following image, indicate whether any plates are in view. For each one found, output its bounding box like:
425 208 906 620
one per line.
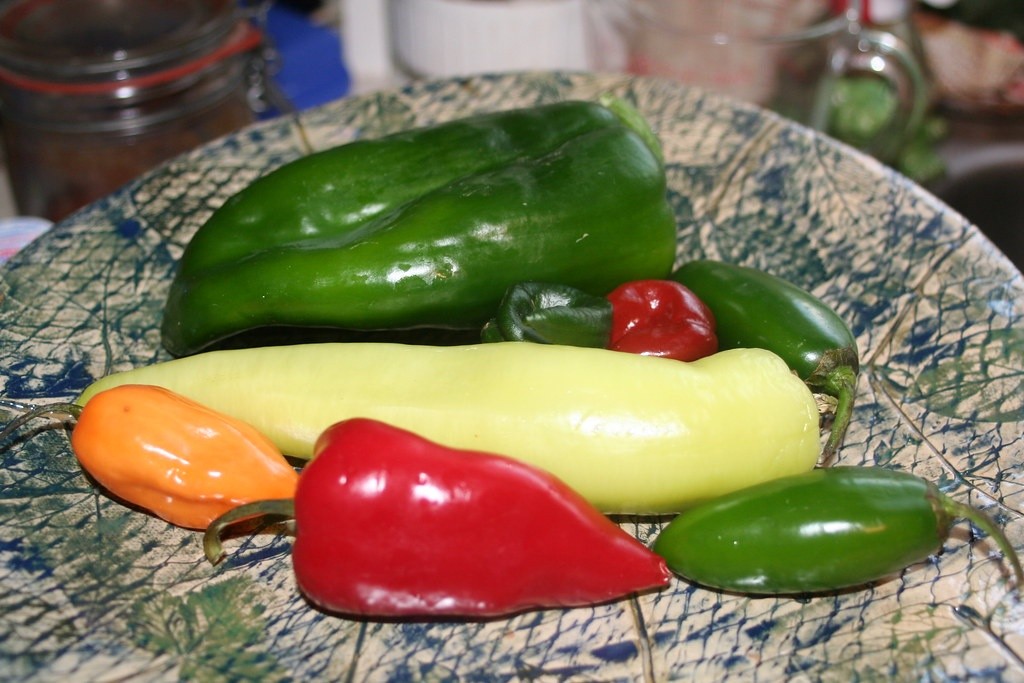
1 65 1024 683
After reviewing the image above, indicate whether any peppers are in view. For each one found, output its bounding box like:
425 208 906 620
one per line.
1 101 1024 618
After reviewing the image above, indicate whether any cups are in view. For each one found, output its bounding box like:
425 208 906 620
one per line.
580 0 917 168
